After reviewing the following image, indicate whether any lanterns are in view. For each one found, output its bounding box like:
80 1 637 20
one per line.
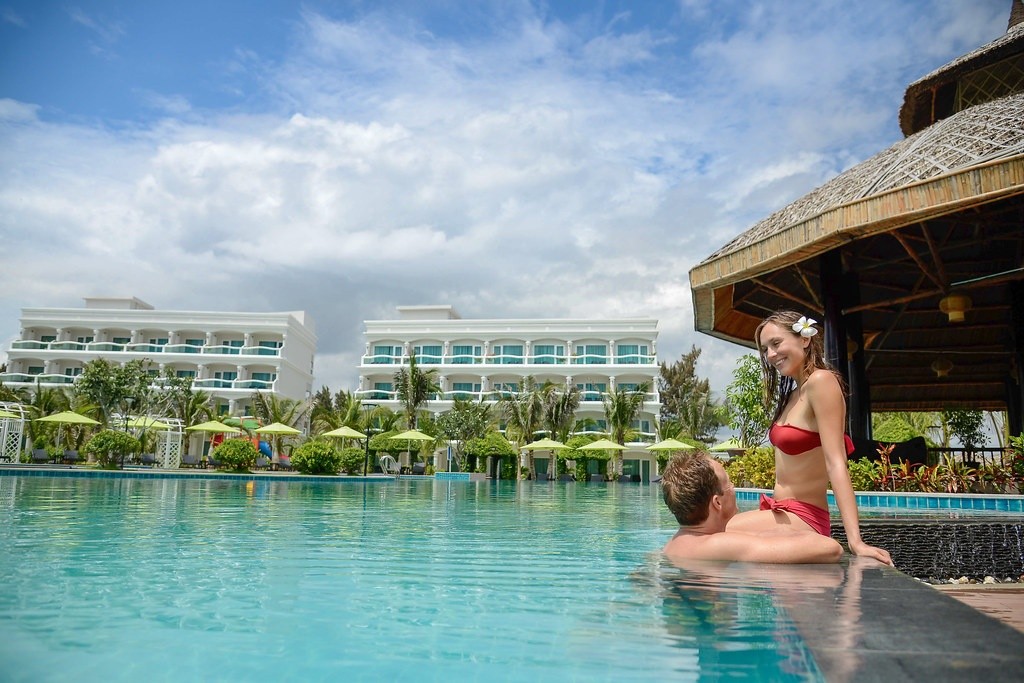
932 359 953 376
939 292 973 321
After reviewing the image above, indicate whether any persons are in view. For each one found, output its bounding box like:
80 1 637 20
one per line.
661 447 844 563
255 422 303 460
725 311 894 566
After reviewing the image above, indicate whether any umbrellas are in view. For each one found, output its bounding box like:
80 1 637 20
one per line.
114 416 173 432
578 438 629 476
0 411 20 420
647 438 695 462
322 426 367 451
35 411 102 463
388 429 434 465
710 438 754 451
520 438 572 478
185 420 242 460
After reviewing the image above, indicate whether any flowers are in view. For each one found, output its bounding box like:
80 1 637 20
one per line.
791 315 818 338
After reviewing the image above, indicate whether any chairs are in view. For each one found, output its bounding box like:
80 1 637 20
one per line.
524 472 642 482
60 450 87 465
412 462 426 474
275 459 292 471
30 448 56 464
386 462 402 475
134 453 161 467
208 455 227 470
254 457 272 470
179 455 202 469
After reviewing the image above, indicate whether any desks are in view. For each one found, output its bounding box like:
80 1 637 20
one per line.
400 466 410 475
270 462 278 471
199 460 208 469
